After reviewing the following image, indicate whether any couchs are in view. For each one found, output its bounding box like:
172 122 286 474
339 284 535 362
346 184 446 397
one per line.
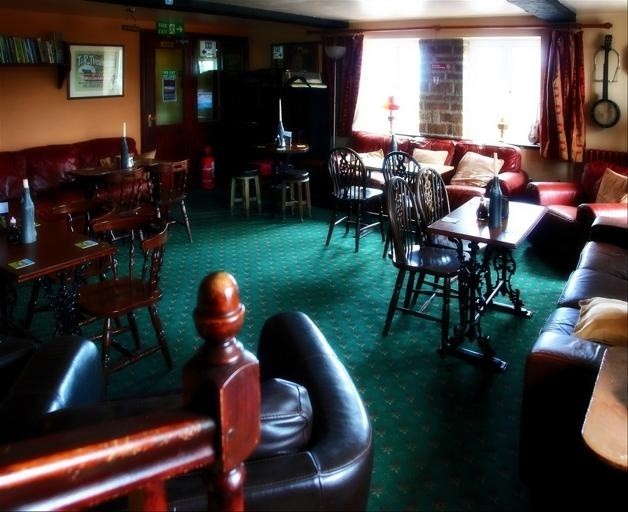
331 138 531 209
31 310 378 508
523 217 626 412
524 156 628 274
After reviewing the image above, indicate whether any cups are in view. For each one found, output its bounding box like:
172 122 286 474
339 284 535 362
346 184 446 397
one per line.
284 130 292 146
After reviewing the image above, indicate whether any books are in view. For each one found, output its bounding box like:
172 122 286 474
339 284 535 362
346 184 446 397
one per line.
0 37 63 65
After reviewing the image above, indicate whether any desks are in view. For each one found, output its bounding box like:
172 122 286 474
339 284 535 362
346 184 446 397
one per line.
245 137 312 176
425 193 549 372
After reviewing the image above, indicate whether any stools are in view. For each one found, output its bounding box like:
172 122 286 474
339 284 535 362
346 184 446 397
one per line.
225 167 265 219
275 169 315 224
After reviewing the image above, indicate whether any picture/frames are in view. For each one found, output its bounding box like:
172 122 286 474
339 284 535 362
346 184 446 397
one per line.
64 41 126 101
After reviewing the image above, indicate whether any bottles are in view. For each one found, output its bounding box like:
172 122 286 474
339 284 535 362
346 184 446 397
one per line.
127 154 134 167
19 188 38 245
489 176 503 229
121 136 129 169
275 121 286 151
476 195 488 222
6 217 21 245
390 134 397 162
502 196 509 220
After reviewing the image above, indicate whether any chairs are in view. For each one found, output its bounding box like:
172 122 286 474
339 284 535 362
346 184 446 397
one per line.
410 166 497 323
2 139 202 405
321 146 386 254
379 177 471 354
380 149 422 261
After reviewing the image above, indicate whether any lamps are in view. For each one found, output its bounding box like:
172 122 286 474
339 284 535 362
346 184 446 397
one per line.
324 45 346 149
382 95 402 133
